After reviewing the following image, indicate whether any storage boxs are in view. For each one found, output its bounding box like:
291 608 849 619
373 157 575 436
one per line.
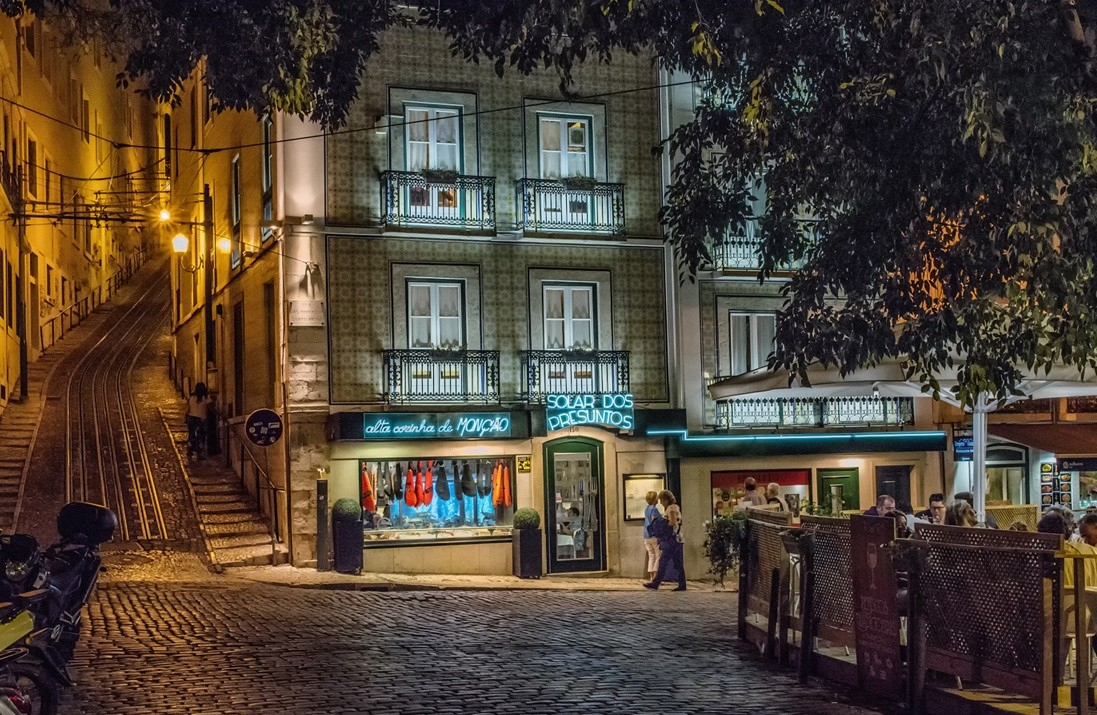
57 501 116 543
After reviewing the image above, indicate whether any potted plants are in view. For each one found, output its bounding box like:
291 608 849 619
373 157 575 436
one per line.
512 507 542 579
420 169 460 184
332 498 364 572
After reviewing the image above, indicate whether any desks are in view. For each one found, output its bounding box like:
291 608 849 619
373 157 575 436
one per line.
557 534 576 559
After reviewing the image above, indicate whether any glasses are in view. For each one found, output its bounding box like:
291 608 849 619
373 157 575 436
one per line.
930 507 944 511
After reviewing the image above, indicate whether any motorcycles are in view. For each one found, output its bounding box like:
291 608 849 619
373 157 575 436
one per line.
0 499 119 715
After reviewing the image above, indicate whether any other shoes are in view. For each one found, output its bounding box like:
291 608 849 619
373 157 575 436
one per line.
643 583 658 591
671 588 685 592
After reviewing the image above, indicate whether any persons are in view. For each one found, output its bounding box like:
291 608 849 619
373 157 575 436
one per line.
862 492 1097 684
560 508 584 544
641 490 687 592
743 477 767 505
186 383 208 459
643 491 664 585
766 483 789 512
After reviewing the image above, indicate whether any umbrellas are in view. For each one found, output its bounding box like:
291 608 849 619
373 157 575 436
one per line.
707 302 1097 528
